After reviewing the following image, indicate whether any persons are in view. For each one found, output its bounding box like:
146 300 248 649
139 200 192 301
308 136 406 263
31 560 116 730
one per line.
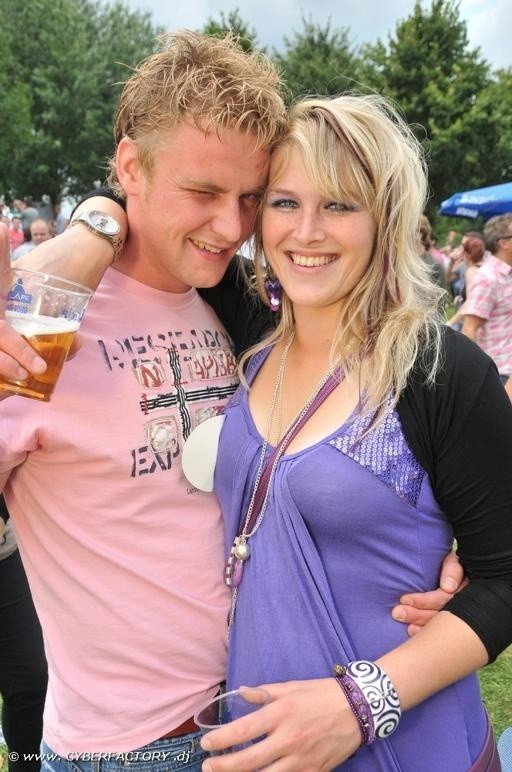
0 28 288 770
8 75 512 772
0 491 42 769
0 214 512 413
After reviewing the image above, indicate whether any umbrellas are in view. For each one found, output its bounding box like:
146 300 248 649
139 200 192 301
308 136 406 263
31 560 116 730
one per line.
433 180 512 222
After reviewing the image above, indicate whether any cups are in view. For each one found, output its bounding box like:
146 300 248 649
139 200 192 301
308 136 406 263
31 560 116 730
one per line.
192 687 272 772
2 269 95 402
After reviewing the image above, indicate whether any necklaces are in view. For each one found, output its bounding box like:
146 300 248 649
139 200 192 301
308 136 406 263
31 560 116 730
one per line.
233 324 377 566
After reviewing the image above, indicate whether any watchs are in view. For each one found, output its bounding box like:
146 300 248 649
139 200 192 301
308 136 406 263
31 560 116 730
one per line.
71 209 125 259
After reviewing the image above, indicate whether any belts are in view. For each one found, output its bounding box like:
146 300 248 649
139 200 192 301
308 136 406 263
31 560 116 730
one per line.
161 701 219 740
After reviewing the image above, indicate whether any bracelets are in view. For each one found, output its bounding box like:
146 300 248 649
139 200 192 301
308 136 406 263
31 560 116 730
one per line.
334 668 375 745
345 662 401 741
